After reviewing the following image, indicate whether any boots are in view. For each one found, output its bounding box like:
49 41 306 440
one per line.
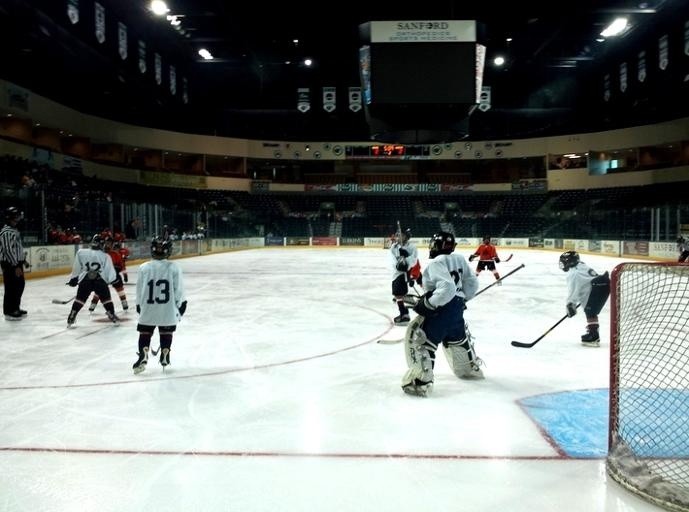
160 335 171 372
496 279 504 287
391 301 410 327
132 336 149 375
120 301 129 314
88 301 97 314
66 307 79 328
5 310 29 323
454 355 483 378
581 325 601 347
402 368 436 398
104 307 121 324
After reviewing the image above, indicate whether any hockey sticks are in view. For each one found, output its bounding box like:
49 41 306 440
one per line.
53 296 75 304
378 264 525 347
470 253 512 262
510 304 583 347
151 345 161 355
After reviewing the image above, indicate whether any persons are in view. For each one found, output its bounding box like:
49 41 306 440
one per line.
113 245 129 283
676 235 689 263
390 228 422 322
18 152 206 242
87 237 129 312
67 234 117 325
560 251 610 342
402 233 478 389
470 234 500 284
133 237 182 372
0 207 29 317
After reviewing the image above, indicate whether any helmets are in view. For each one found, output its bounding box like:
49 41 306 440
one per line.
150 237 173 260
104 236 114 250
119 249 129 256
427 232 457 258
6 208 25 227
91 234 102 252
397 228 412 244
483 234 492 244
558 250 580 270
